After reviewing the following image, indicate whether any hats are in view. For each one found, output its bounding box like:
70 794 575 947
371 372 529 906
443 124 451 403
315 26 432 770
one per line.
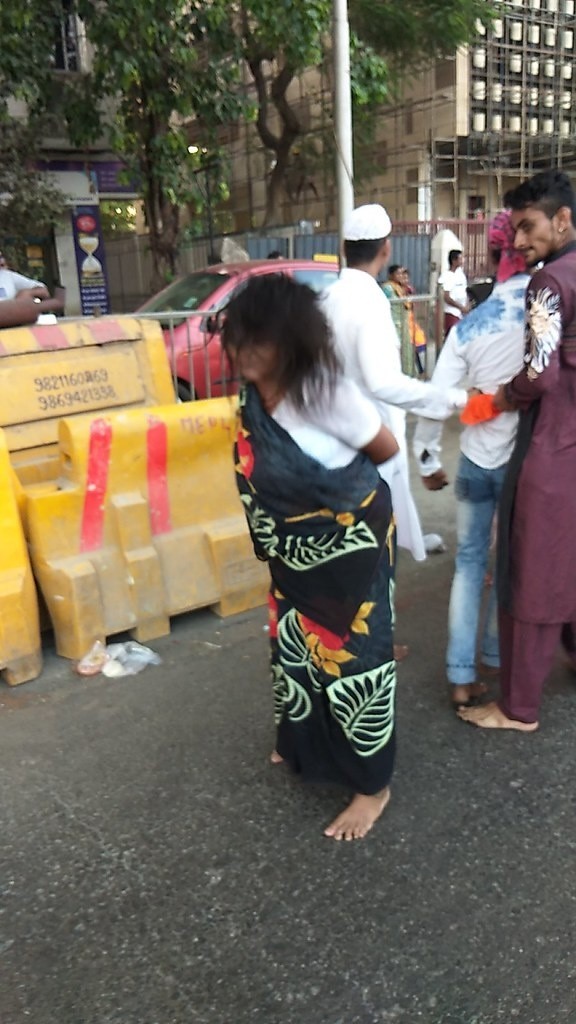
342 203 392 241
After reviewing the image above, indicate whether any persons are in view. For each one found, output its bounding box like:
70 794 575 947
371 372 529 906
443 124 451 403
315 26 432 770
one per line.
313 204 480 653
380 169 576 734
266 250 285 260
0 248 59 329
217 273 402 842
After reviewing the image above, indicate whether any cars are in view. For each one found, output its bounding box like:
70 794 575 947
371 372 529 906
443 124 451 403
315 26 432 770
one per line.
131 261 340 402
